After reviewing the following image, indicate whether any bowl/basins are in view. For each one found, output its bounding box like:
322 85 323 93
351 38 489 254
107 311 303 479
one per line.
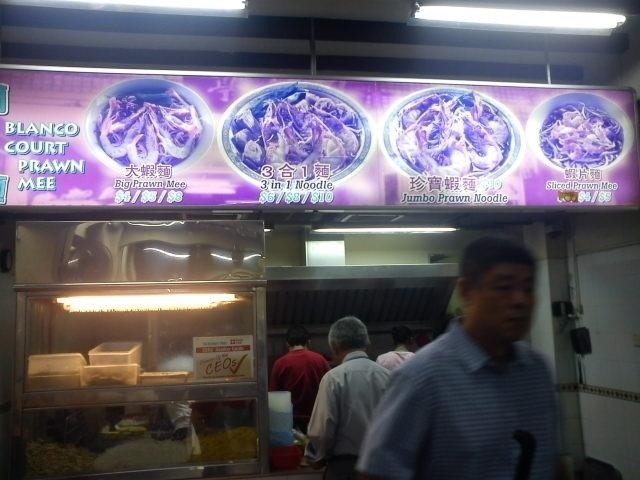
212 82 375 190
79 76 215 178
525 93 634 176
268 441 307 471
373 87 525 194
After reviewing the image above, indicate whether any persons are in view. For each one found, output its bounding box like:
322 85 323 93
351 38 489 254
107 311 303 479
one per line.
355 234 569 479
270 325 333 432
303 315 394 480
375 324 416 370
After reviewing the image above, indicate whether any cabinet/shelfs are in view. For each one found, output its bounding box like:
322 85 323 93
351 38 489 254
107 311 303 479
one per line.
4 210 267 479
